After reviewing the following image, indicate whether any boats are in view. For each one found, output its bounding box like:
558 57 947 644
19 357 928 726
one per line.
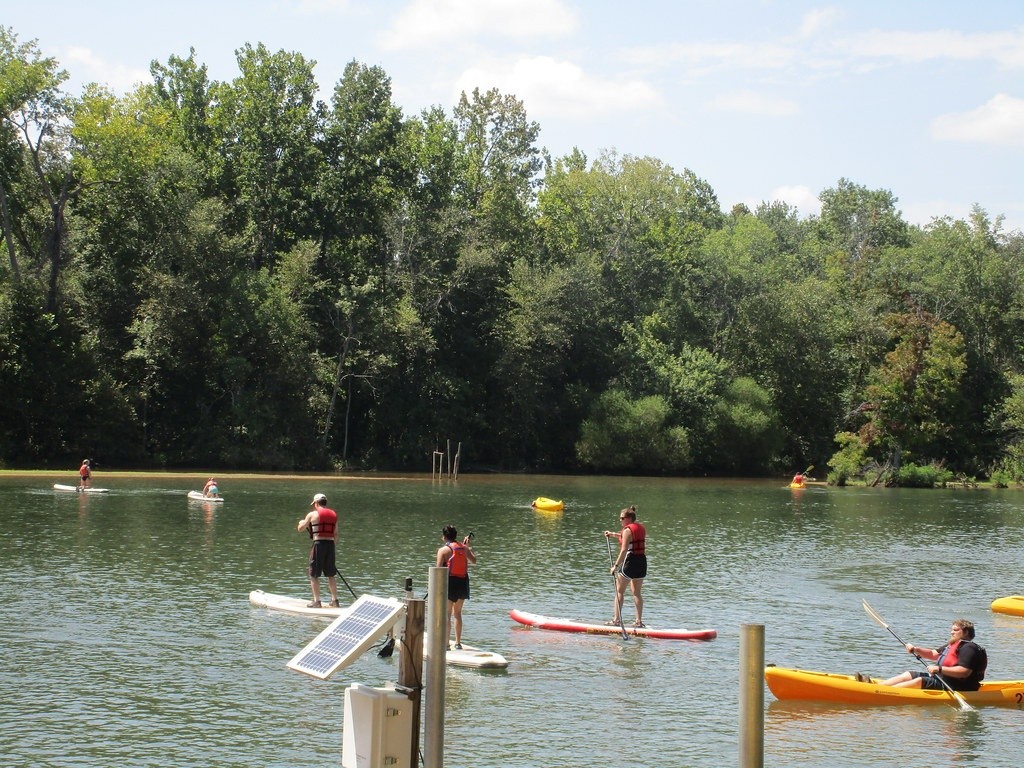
990 595 1024 618
763 662 1024 707
532 496 563 510
790 480 806 488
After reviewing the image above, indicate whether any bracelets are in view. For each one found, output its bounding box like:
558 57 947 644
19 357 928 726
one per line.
938 664 942 674
614 564 619 567
613 532 615 537
469 547 472 550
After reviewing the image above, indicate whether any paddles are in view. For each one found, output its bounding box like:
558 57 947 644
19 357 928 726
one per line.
861 598 972 711
377 532 475 657
788 465 814 486
605 531 628 641
304 524 359 602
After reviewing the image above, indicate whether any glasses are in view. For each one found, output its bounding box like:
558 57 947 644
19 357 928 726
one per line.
620 516 630 520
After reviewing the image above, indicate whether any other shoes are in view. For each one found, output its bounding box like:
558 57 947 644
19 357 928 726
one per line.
854 672 862 682
446 644 452 652
632 621 643 627
328 599 341 608
307 600 322 609
864 674 872 683
454 642 463 650
604 620 621 626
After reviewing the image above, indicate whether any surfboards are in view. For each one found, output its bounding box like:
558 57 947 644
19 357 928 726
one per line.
510 608 716 640
395 630 508 668
188 490 224 502
248 589 351 617
54 483 110 492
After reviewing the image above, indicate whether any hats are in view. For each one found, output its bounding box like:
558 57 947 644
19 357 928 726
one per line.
310 493 327 506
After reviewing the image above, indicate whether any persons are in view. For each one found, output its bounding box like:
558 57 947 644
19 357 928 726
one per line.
605 506 648 629
202 477 219 499
854 618 981 691
297 492 340 608
792 473 802 484
435 526 478 652
76 459 93 493
